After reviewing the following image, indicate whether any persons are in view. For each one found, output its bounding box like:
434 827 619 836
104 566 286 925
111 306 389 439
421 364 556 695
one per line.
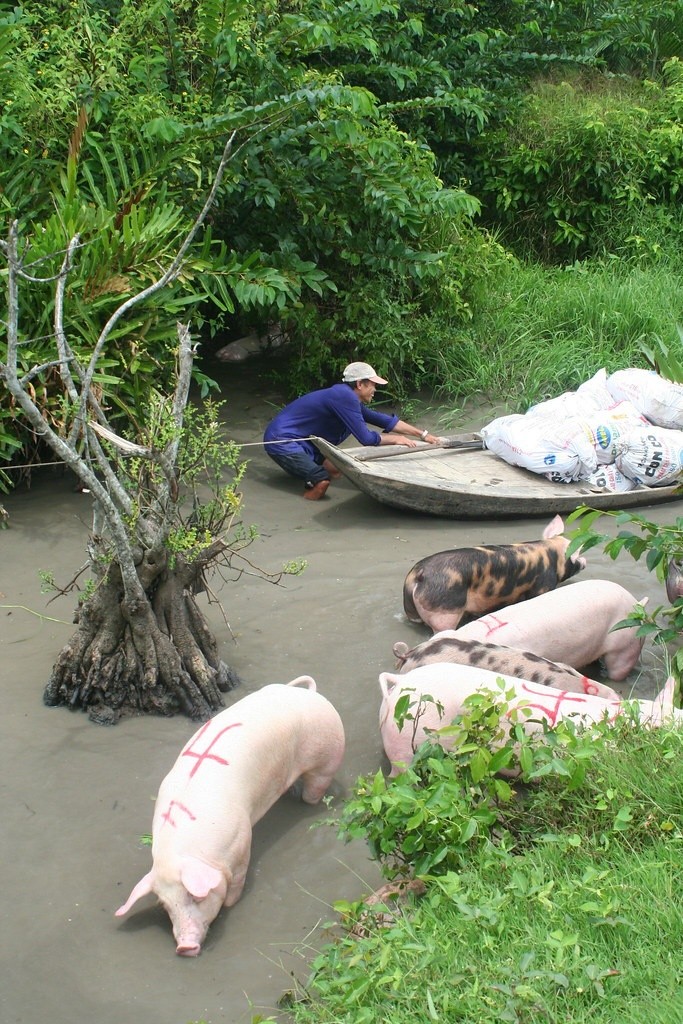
263 362 440 500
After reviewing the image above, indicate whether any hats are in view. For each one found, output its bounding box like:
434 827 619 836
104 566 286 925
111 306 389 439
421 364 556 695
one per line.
342 361 389 385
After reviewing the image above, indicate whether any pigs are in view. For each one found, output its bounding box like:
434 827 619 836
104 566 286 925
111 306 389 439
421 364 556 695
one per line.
379 515 683 784
115 674 346 958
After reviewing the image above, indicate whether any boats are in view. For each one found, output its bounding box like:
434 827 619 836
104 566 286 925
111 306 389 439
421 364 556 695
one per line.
309 424 683 522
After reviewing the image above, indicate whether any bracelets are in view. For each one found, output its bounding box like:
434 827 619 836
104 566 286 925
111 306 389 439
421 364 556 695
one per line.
420 429 429 439
